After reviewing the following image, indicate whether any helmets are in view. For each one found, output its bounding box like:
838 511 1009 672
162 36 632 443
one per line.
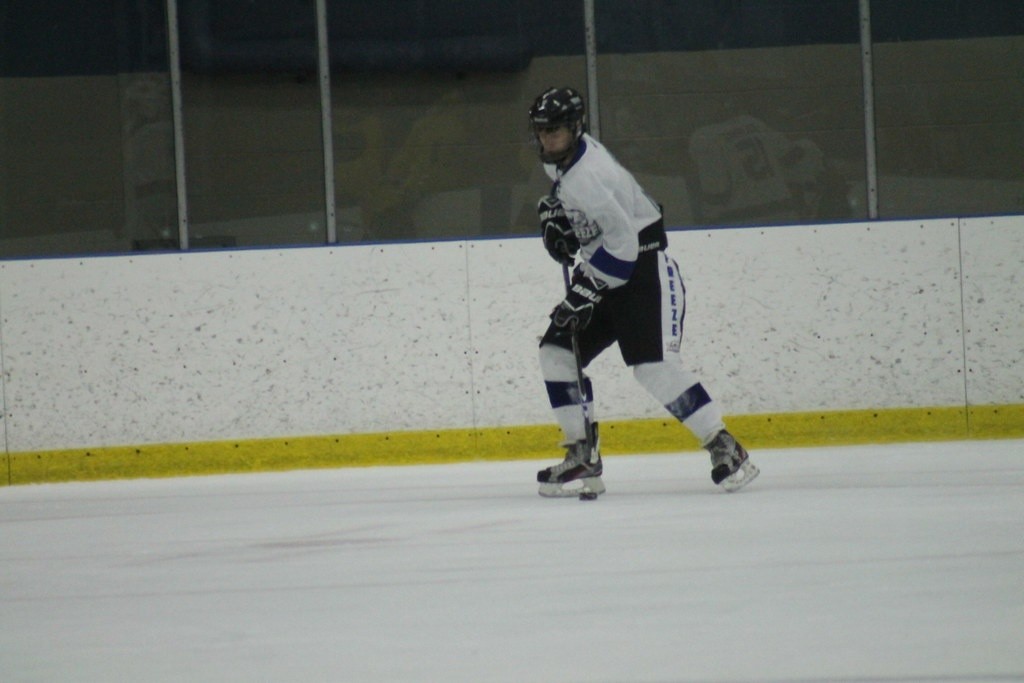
528 87 587 162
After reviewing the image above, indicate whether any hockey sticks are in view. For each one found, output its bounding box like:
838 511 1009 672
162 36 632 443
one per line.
561 257 599 502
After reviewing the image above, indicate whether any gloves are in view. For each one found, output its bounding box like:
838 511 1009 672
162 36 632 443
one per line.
554 278 608 341
537 197 580 266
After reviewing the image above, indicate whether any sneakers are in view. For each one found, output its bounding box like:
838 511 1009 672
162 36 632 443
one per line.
537 422 606 497
702 428 760 492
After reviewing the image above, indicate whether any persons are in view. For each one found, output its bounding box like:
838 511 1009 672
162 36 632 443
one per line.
686 89 849 226
527 87 760 501
